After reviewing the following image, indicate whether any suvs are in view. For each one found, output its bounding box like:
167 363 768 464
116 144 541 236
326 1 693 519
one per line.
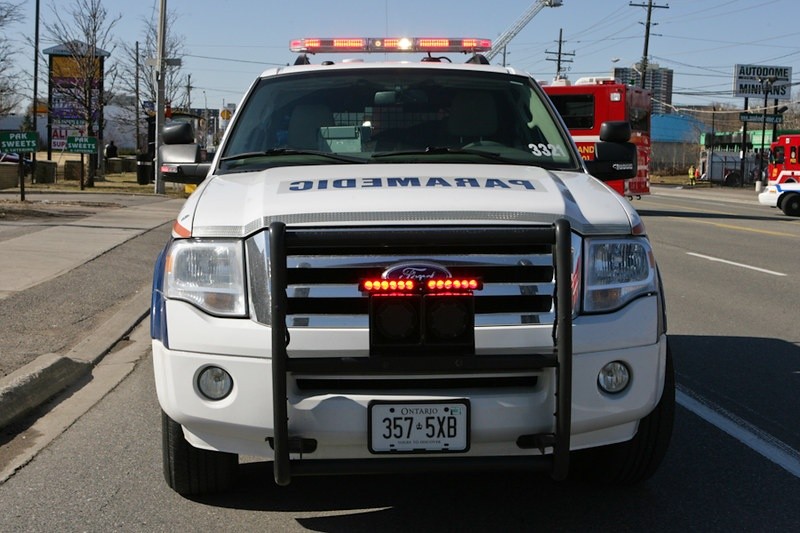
150 35 677 502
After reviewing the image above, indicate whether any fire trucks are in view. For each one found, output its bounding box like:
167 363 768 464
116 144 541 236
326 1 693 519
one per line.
481 0 653 201
766 133 800 188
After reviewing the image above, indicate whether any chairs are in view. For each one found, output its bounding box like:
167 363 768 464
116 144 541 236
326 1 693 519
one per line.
450 91 499 144
286 107 337 153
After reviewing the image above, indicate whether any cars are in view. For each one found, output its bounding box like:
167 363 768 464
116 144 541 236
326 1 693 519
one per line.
758 182 800 216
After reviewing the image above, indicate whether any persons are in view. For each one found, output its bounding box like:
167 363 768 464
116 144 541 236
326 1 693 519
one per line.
107 141 118 157
103 144 109 157
689 165 697 186
